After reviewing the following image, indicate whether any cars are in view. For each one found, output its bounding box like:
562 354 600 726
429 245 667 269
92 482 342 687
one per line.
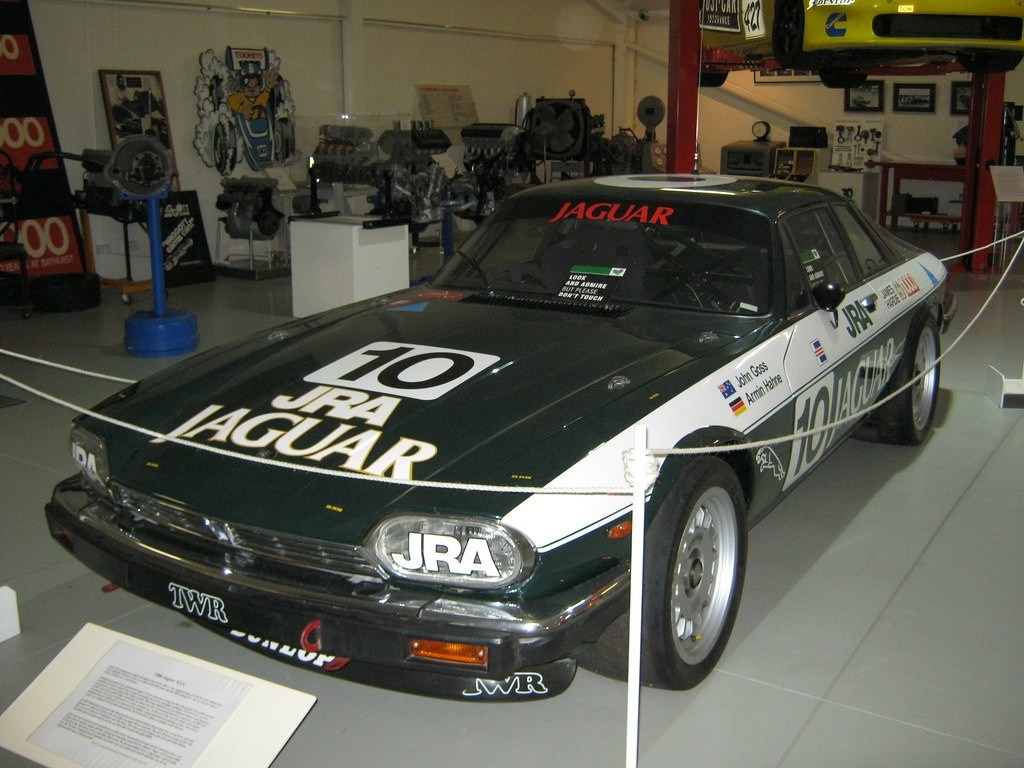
42 170 960 704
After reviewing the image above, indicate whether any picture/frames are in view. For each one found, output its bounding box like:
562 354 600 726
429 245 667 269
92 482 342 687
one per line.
844 80 884 112
892 83 935 112
950 81 970 114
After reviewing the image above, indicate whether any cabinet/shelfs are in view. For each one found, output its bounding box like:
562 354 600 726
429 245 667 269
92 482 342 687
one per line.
864 159 965 234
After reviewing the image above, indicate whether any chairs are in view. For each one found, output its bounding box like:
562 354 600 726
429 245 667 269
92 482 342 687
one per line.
516 222 828 318
